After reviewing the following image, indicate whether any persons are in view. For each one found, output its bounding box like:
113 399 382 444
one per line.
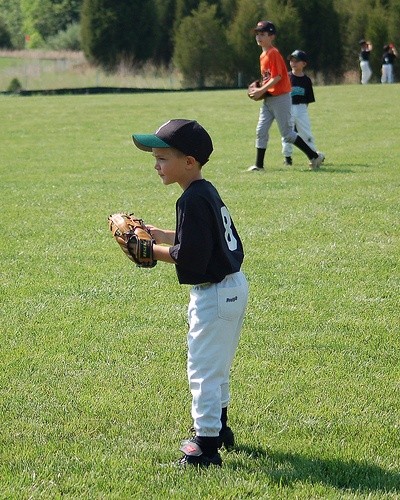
380 43 397 84
359 39 372 85
107 119 248 468
281 49 325 166
245 20 324 171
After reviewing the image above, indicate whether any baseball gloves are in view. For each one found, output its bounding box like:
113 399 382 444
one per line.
249 80 267 101
108 214 158 268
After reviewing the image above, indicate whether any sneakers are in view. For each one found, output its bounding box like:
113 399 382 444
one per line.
192 427 234 448
249 166 263 170
177 442 222 467
308 152 324 170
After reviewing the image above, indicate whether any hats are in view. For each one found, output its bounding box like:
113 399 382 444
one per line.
358 40 366 45
286 50 307 60
254 21 275 33
383 45 390 51
132 119 213 165
